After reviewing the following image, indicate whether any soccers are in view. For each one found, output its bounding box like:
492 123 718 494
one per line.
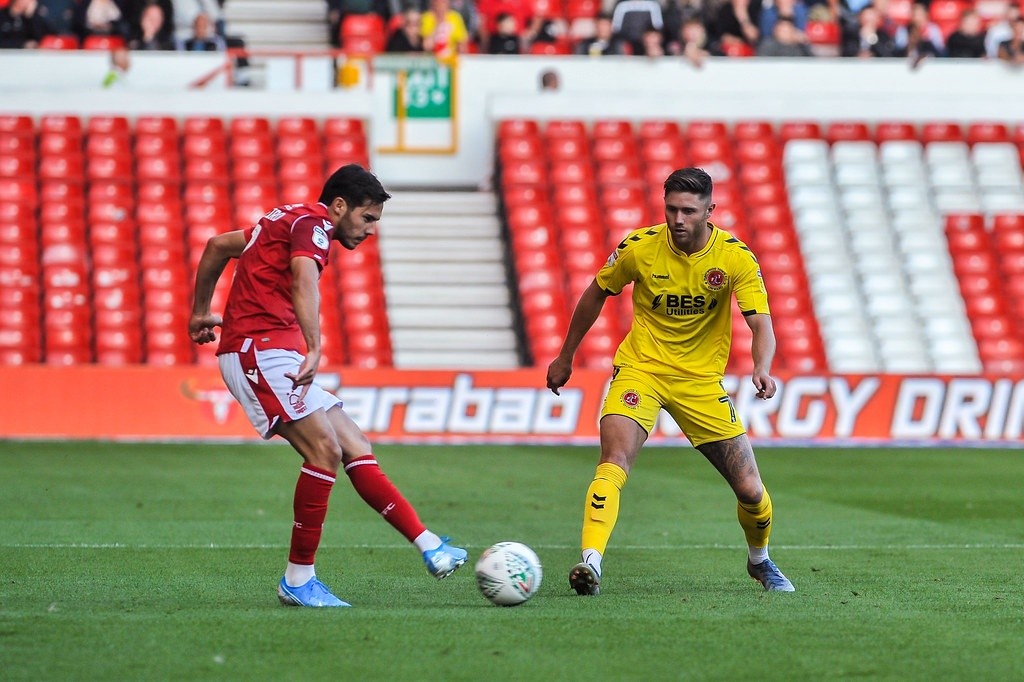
474 541 543 608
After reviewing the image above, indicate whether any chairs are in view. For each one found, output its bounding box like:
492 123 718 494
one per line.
0 0 1024 383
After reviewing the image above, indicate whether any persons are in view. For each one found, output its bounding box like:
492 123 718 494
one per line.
0 0 217 52
546 167 795 594
326 0 1024 71
187 165 468 609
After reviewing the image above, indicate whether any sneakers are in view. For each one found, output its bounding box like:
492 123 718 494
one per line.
277 576 352 608
747 555 795 592
423 537 467 580
569 563 600 596
